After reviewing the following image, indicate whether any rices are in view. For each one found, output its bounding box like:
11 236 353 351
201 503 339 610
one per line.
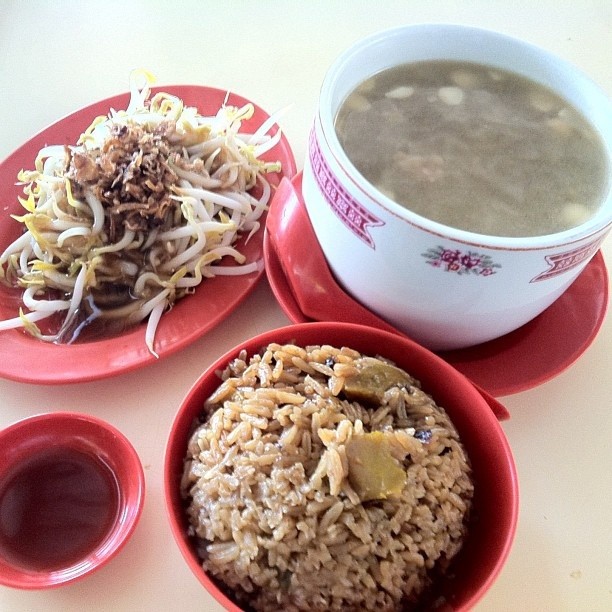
177 341 479 607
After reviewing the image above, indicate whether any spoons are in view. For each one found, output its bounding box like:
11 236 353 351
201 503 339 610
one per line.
266 176 511 421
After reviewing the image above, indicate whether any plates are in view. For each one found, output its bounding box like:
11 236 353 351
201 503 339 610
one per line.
0 85 296 383
262 169 608 398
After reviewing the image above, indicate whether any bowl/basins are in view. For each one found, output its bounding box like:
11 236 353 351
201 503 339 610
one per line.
300 25 612 351
0 412 147 589
165 322 518 611
